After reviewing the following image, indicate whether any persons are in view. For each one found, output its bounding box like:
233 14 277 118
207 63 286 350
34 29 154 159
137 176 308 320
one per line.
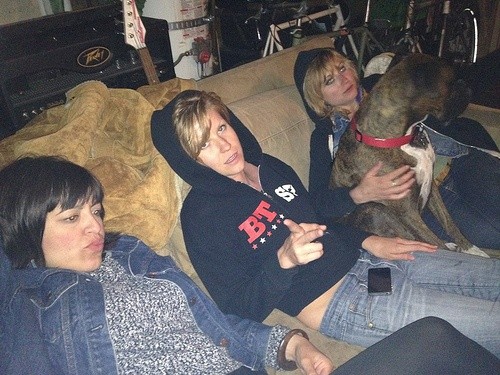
150 89 500 358
294 47 500 251
0 152 500 375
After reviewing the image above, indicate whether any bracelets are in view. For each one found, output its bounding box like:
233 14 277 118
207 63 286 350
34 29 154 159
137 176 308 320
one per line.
277 329 310 371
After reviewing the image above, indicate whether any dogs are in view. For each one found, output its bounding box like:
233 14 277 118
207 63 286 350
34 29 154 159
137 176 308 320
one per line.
326 42 492 261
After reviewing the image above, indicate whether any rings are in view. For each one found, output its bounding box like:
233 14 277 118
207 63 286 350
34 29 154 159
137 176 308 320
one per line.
392 181 398 186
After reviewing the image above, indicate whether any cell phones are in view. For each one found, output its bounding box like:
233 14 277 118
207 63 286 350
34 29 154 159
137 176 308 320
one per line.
368 267 392 296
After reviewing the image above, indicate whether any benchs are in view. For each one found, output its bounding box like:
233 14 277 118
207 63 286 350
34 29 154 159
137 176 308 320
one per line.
149 34 500 375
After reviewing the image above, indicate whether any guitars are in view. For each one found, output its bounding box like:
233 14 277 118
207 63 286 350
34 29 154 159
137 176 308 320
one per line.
120 0 161 86
